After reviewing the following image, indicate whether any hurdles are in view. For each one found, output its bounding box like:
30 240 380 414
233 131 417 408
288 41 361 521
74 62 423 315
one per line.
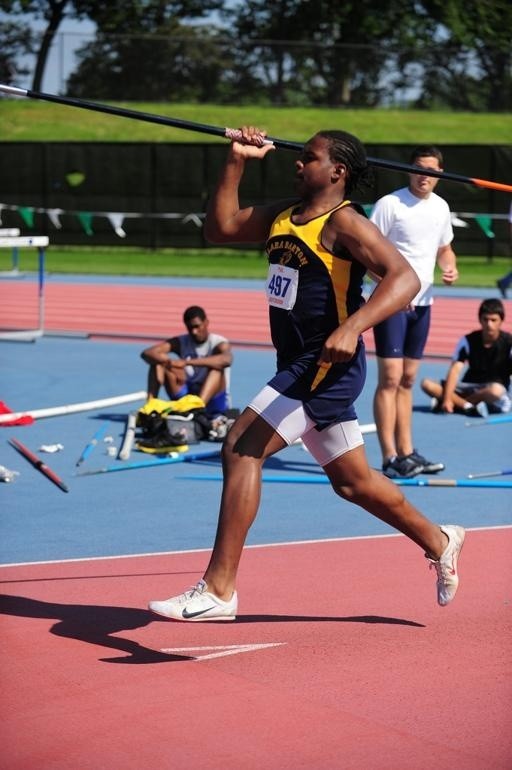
1 228 51 340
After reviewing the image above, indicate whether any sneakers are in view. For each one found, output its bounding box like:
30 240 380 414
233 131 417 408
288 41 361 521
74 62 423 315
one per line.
149 580 238 622
136 429 190 454
382 455 424 478
425 525 465 606
404 449 445 474
467 401 487 417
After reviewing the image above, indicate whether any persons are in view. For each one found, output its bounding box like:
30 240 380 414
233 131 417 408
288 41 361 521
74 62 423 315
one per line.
420 297 512 418
147 126 466 624
366 144 458 479
136 306 233 426
